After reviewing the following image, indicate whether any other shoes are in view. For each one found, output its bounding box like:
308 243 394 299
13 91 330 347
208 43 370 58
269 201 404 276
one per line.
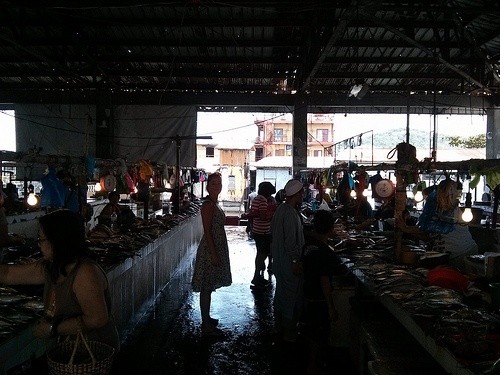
283 335 296 342
202 318 218 323
252 275 260 284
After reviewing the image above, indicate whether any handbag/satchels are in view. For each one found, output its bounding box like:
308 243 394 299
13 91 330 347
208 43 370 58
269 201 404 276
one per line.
74 191 93 223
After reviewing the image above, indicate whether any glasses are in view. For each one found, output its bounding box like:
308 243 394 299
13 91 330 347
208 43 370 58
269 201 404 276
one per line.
36 233 48 245
7 190 17 194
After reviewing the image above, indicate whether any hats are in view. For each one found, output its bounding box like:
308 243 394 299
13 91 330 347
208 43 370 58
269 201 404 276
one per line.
285 180 303 196
2 184 17 193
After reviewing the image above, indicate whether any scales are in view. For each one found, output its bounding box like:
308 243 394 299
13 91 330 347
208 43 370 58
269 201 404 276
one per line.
94 175 116 229
370 177 397 237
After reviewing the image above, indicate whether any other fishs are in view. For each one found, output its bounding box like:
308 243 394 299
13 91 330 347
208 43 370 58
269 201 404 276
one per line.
337 246 500 375
0 211 189 341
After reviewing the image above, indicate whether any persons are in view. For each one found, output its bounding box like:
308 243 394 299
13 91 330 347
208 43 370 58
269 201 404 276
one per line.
341 186 410 221
393 178 479 273
0 211 120 375
100 191 122 215
247 179 338 375
191 172 232 336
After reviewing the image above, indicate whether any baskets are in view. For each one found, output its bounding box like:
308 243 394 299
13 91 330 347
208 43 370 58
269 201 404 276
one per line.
47 318 115 375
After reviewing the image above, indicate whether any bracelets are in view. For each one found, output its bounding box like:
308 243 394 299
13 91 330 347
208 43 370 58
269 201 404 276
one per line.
50 321 54 335
